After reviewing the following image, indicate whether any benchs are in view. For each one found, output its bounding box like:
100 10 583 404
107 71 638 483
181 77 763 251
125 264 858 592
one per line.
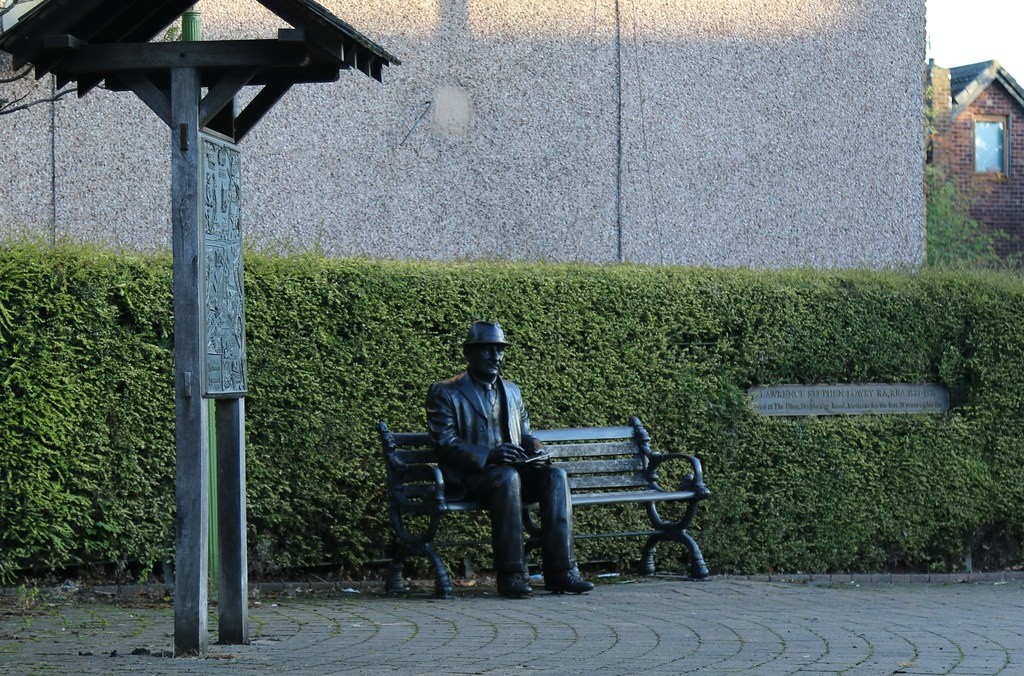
373 417 712 601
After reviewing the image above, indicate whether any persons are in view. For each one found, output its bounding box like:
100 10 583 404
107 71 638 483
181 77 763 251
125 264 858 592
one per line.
425 321 595 599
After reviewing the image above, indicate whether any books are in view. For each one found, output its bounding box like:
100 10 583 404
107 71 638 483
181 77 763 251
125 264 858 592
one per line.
515 450 553 463
